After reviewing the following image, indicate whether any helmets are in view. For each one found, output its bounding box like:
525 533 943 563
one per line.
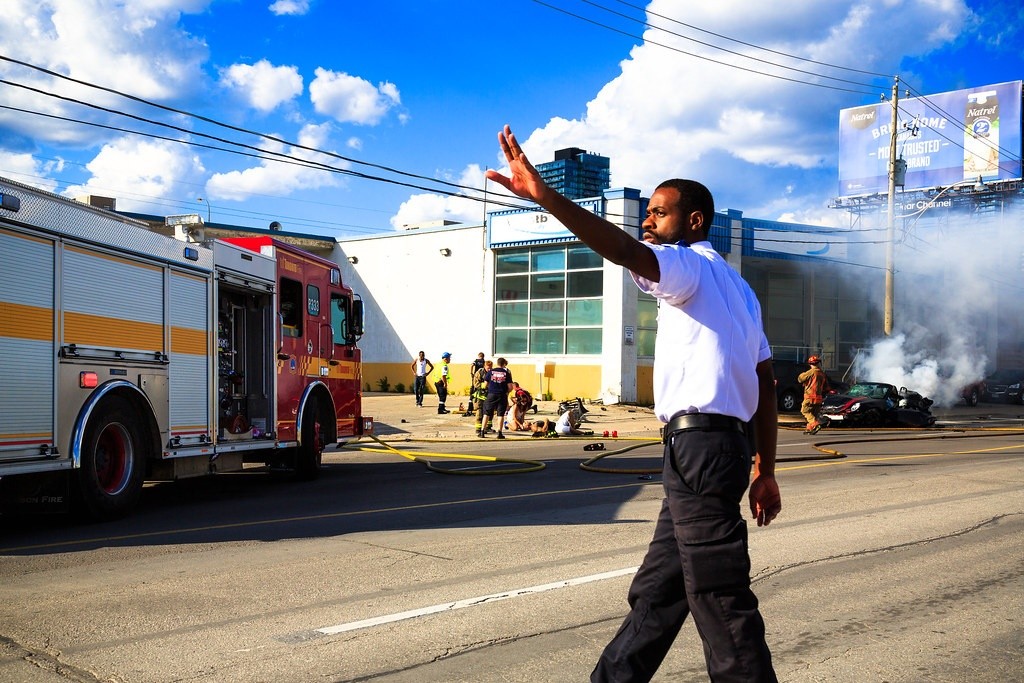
441 352 451 358
807 356 819 365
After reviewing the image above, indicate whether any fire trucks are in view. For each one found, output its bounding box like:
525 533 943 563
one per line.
0 174 374 521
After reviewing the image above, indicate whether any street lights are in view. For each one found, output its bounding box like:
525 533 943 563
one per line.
883 173 986 339
197 198 211 224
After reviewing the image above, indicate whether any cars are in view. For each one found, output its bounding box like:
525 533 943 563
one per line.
947 371 1024 407
767 359 907 429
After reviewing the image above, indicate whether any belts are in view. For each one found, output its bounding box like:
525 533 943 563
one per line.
661 413 743 445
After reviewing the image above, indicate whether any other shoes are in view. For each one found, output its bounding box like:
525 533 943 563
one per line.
478 433 484 438
811 425 821 435
497 434 504 439
802 430 814 435
416 402 422 408
485 426 495 433
462 411 472 417
438 408 450 414
476 429 488 435
533 405 537 415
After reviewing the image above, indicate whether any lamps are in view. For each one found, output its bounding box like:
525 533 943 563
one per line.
975 174 985 191
348 257 357 264
440 248 451 255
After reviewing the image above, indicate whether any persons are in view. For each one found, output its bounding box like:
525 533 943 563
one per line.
411 351 434 407
478 358 513 439
504 382 555 436
798 356 825 435
473 361 496 433
433 352 452 414
462 352 485 417
555 409 594 435
486 124 782 683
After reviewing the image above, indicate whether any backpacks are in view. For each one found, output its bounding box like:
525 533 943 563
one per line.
515 388 533 410
